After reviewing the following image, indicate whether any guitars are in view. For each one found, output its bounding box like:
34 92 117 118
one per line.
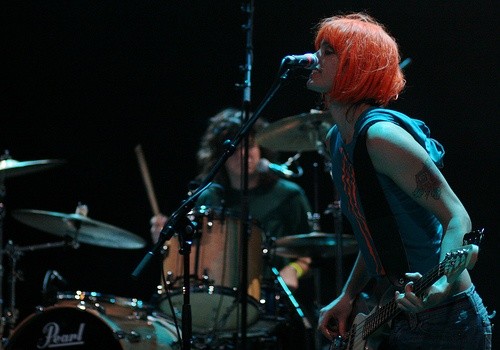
328 228 485 350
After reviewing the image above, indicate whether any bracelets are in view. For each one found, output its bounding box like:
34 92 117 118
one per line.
290 262 304 278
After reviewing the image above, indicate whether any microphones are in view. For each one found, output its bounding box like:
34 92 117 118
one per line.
285 53 319 69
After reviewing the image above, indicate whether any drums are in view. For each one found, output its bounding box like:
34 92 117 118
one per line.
4 293 183 350
102 296 155 318
160 204 265 332
56 291 103 306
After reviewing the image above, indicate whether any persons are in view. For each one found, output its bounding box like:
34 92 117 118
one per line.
305 12 496 350
148 110 320 350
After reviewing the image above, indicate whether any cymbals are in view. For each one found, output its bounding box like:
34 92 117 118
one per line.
252 109 334 152
15 208 147 250
262 232 362 259
0 155 74 175
